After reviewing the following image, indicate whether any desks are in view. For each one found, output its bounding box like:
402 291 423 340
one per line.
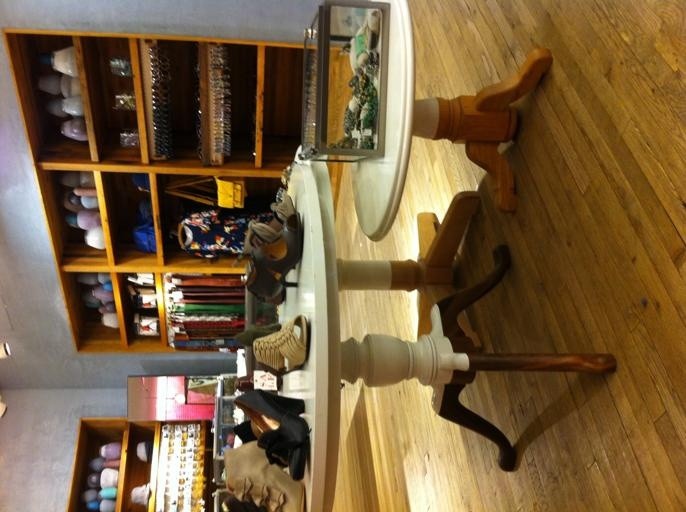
232 0 616 510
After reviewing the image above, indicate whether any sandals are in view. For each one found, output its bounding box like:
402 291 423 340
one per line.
250 312 310 379
240 188 298 257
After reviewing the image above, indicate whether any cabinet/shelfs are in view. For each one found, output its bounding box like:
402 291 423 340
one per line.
66 416 208 512
4 29 352 354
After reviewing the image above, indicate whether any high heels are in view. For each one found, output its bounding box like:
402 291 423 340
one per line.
232 384 316 483
239 209 321 299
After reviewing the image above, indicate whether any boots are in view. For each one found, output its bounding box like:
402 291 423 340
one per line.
211 437 308 512
